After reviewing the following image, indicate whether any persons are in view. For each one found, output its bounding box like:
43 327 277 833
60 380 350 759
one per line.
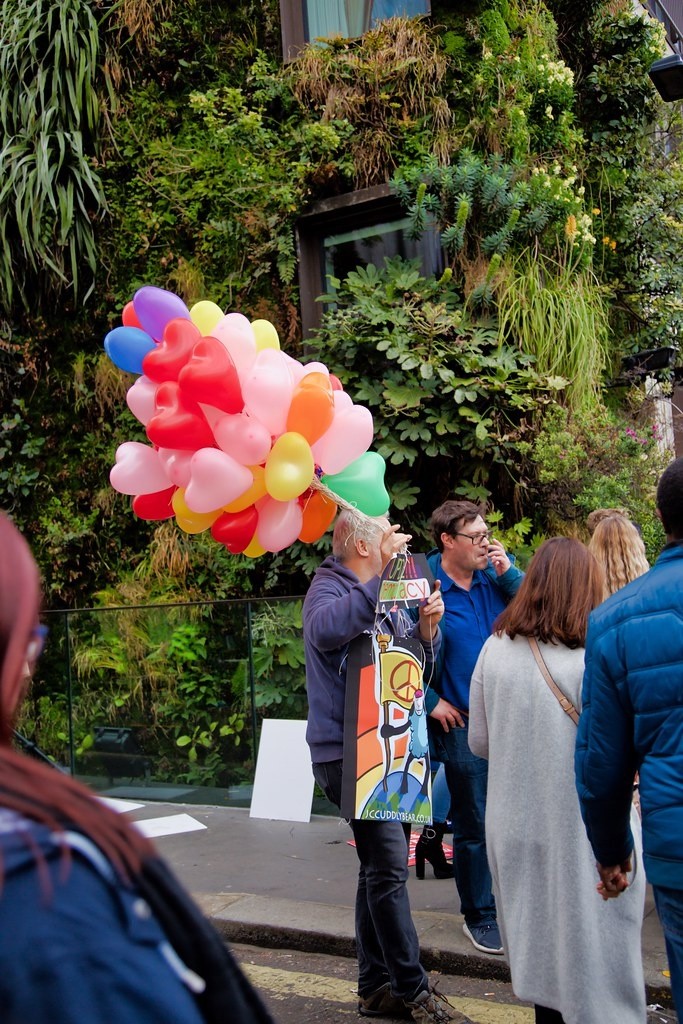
300 511 477 1024
0 504 279 1024
402 762 455 882
574 460 683 1024
426 500 525 954
467 536 647 1024
586 506 653 605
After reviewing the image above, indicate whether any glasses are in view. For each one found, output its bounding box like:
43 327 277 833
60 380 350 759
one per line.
457 531 491 545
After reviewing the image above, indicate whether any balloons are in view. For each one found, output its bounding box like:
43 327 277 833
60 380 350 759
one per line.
103 287 392 560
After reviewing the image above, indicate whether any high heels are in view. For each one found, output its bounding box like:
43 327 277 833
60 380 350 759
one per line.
414 824 455 878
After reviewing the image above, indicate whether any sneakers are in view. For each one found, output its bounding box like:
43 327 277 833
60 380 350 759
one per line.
402 981 472 1024
358 982 402 1015
462 922 505 954
